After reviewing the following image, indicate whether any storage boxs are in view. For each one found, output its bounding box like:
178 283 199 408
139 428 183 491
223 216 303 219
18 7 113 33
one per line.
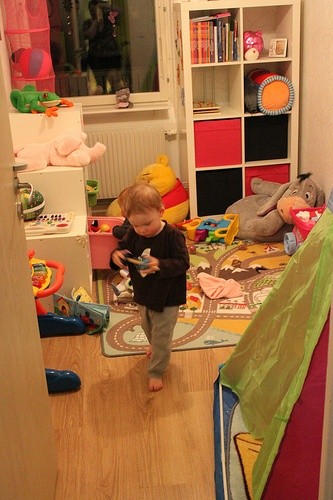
194 121 243 167
246 165 288 196
196 171 243 218
245 117 289 162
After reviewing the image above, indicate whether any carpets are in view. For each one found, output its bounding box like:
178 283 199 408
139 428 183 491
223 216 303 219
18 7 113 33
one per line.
95 237 292 363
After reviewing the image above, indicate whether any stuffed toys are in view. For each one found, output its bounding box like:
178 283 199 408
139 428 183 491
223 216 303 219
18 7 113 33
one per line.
225 172 324 241
105 154 190 225
10 85 73 116
15 131 109 171
117 88 133 109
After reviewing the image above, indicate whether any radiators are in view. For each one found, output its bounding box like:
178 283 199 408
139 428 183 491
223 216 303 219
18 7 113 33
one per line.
87 123 179 203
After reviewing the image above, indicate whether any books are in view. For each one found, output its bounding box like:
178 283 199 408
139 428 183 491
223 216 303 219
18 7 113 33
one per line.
190 12 239 64
193 101 222 114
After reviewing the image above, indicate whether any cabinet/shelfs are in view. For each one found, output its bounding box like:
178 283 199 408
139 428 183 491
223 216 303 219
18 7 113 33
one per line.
174 0 301 220
10 101 95 310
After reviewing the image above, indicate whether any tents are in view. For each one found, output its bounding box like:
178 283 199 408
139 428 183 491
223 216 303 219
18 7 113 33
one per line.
211 189 332 500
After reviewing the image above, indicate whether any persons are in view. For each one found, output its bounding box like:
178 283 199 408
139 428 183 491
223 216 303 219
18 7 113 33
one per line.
109 184 191 389
79 0 125 94
